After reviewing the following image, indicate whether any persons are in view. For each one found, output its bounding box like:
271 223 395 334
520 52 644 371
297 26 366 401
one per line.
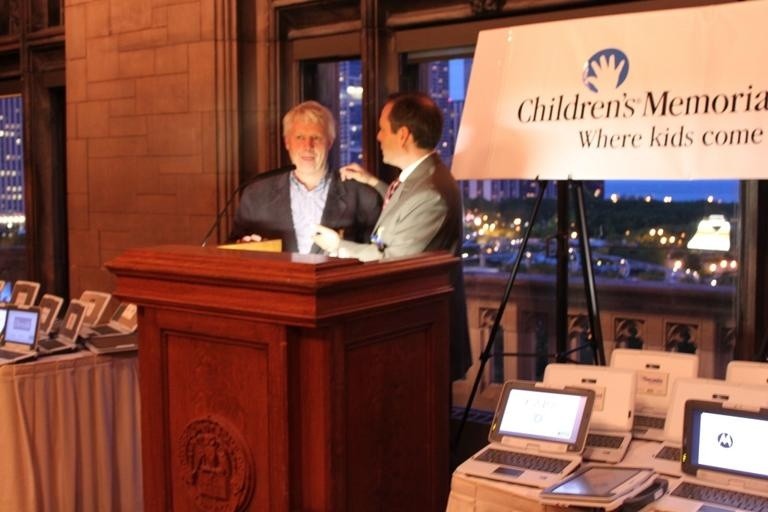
308 93 475 386
221 98 383 262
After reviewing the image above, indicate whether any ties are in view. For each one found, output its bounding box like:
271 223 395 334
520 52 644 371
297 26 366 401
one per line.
381 178 400 207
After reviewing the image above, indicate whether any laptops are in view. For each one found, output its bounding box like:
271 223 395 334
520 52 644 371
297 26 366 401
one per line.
0 279 138 368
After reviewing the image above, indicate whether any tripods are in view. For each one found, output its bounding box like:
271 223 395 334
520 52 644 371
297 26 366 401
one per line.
451 179 606 465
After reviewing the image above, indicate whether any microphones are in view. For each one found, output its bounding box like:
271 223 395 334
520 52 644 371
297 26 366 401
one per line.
198 164 298 245
